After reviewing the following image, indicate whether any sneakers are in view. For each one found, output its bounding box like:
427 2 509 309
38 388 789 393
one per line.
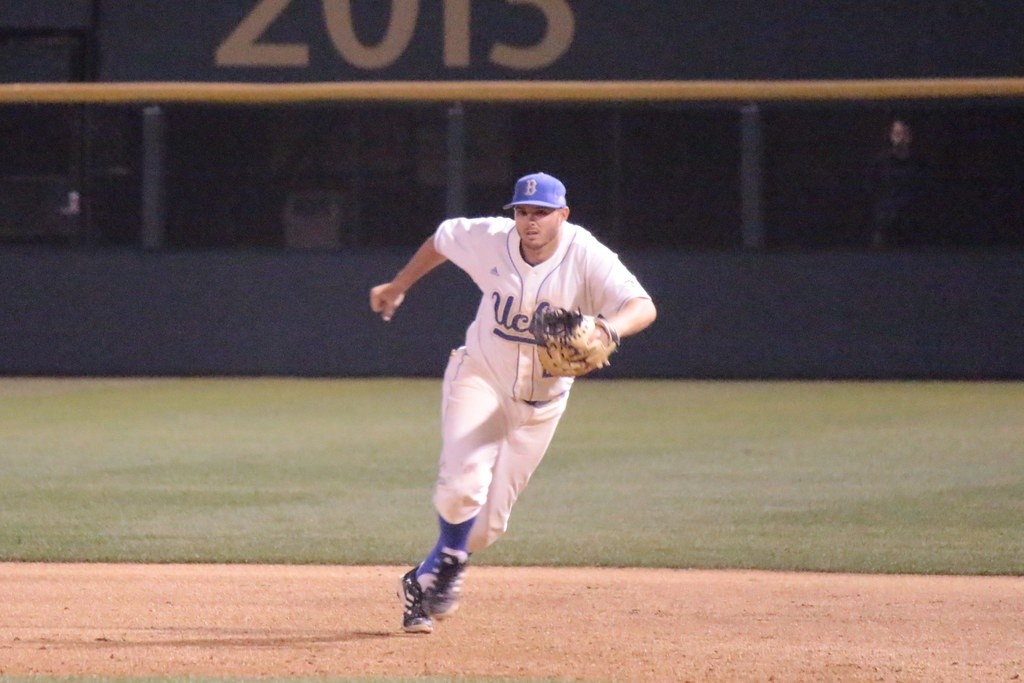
397 566 434 633
425 546 472 621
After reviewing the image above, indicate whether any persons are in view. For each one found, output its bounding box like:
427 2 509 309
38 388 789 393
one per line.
869 117 942 247
366 171 659 633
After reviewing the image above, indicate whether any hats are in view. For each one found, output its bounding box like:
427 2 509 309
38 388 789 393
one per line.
502 172 567 209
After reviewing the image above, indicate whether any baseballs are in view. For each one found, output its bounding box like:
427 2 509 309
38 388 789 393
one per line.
580 314 594 337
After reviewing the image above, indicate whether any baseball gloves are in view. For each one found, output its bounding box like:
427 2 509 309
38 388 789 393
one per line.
529 307 617 378
